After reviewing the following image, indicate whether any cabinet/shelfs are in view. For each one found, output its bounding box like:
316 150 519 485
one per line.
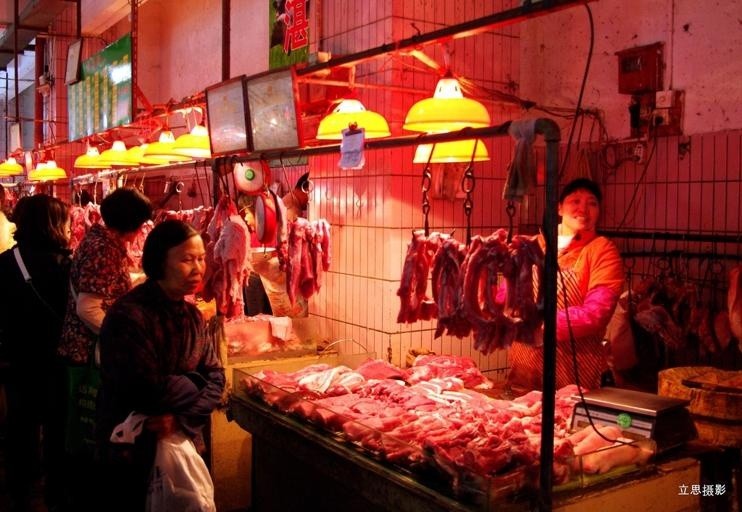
207 336 702 512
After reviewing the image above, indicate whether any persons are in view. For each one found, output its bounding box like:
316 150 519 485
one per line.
0 182 17 254
0 195 84 511
75 216 224 510
53 185 153 398
504 174 638 395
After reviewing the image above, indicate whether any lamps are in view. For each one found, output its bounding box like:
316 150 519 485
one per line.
0 39 491 178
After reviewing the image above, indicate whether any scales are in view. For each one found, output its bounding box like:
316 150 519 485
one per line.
569 386 699 456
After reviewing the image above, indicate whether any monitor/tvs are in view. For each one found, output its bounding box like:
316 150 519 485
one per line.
204 73 252 158
242 65 306 155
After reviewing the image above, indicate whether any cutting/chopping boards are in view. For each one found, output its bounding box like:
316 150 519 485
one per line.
657 366 742 448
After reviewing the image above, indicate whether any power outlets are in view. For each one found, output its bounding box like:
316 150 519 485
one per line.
652 108 669 126
